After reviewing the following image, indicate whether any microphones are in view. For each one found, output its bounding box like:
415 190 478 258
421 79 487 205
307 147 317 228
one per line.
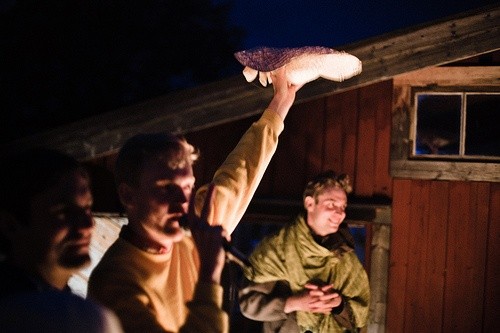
177 212 254 271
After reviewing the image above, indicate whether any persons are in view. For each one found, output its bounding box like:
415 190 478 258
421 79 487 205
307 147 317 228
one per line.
238 170 371 333
86 66 302 333
0 150 124 333
